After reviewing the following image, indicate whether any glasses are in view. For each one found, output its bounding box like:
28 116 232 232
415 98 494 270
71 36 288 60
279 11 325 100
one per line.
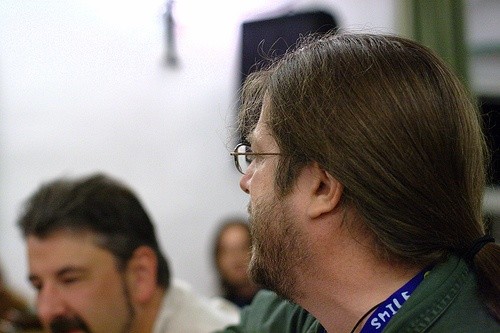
232 143 304 173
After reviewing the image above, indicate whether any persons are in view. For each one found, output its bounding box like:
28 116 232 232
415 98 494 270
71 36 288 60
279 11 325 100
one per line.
228 34 500 333
0 173 260 333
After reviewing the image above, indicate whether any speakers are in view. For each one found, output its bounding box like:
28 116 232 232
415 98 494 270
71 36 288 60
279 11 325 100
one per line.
240 9 338 147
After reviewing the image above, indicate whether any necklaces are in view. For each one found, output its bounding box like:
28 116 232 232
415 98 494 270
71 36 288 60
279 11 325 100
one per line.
351 300 386 333
